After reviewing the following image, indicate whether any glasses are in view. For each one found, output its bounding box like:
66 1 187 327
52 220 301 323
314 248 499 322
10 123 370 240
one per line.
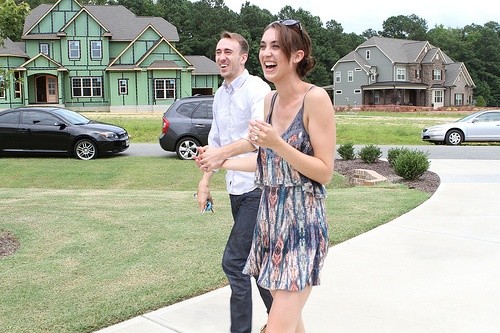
264 20 306 47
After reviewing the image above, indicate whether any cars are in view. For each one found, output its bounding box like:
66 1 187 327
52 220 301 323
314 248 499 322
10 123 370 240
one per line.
158 94 214 159
0 106 130 161
422 110 500 146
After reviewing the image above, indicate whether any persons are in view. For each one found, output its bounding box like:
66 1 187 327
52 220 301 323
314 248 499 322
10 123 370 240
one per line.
192 20 336 333
197 30 271 333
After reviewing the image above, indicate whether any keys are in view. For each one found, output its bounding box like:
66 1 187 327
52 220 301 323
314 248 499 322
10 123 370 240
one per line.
194 193 214 214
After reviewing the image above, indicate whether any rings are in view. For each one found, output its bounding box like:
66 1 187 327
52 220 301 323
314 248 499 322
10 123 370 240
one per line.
255 135 258 141
